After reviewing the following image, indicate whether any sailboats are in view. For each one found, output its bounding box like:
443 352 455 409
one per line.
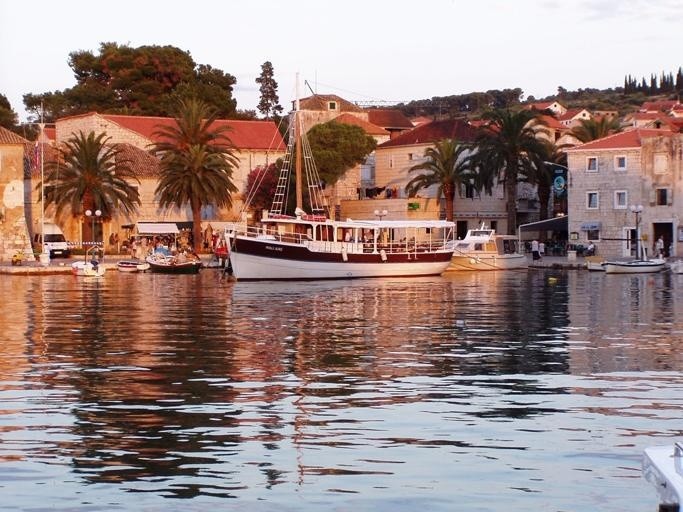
212 71 334 259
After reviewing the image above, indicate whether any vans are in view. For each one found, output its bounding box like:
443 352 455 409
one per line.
32 223 69 259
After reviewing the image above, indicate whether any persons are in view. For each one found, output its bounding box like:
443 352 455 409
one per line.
402 237 408 248
530 238 546 264
345 232 384 253
653 235 673 258
96 231 102 242
582 241 595 257
410 237 415 247
110 224 193 253
12 252 24 265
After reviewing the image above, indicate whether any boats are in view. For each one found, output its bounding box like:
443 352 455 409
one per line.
145 251 203 274
435 221 528 272
116 260 151 272
600 259 667 274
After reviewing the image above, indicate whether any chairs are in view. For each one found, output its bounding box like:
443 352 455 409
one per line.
565 244 596 256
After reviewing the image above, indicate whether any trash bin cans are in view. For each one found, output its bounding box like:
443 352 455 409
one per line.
568 251 577 262
40 254 49 265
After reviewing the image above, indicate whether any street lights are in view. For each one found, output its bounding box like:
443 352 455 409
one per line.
373 209 387 220
542 161 572 250
85 209 103 247
630 204 646 261
